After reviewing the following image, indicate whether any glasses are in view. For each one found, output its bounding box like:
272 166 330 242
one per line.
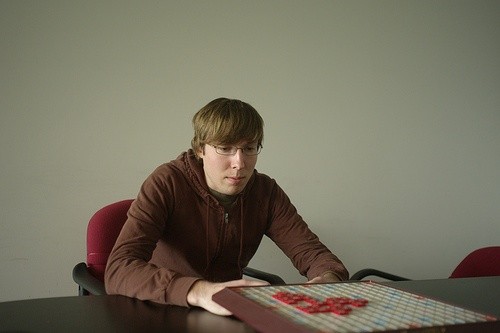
208 143 263 155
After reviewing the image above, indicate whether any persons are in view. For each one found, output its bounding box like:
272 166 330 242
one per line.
104 97 351 315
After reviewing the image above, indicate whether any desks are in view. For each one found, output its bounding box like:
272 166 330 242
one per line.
0 277 500 333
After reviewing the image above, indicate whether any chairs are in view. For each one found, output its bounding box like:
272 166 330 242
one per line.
349 246 500 282
72 199 286 295
